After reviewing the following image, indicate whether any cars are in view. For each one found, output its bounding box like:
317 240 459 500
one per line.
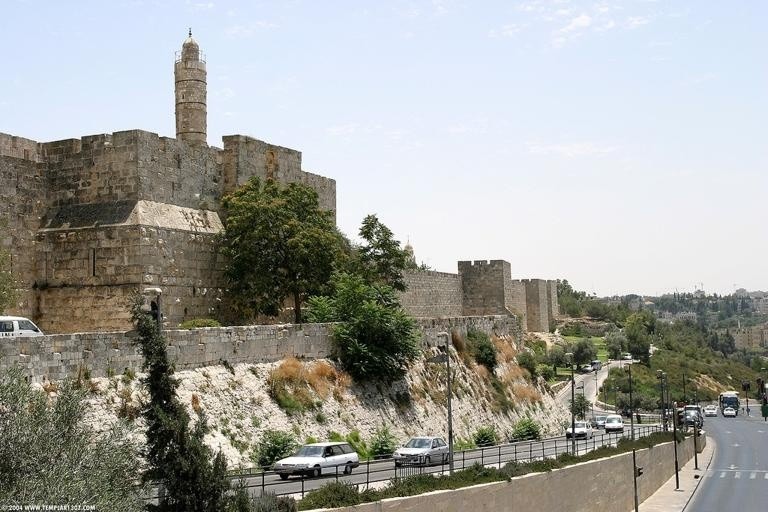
273 442 360 480
591 416 606 429
624 353 632 360
582 361 602 372
566 422 592 440
683 404 736 428
605 415 624 433
392 437 449 467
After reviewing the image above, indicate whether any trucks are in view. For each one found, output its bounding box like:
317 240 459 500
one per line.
718 391 740 416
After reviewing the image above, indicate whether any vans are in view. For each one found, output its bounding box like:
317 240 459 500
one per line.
0 316 45 337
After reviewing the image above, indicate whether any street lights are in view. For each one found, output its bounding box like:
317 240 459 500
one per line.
581 380 586 421
436 332 454 476
611 378 618 414
657 370 671 432
565 353 580 456
624 362 635 440
